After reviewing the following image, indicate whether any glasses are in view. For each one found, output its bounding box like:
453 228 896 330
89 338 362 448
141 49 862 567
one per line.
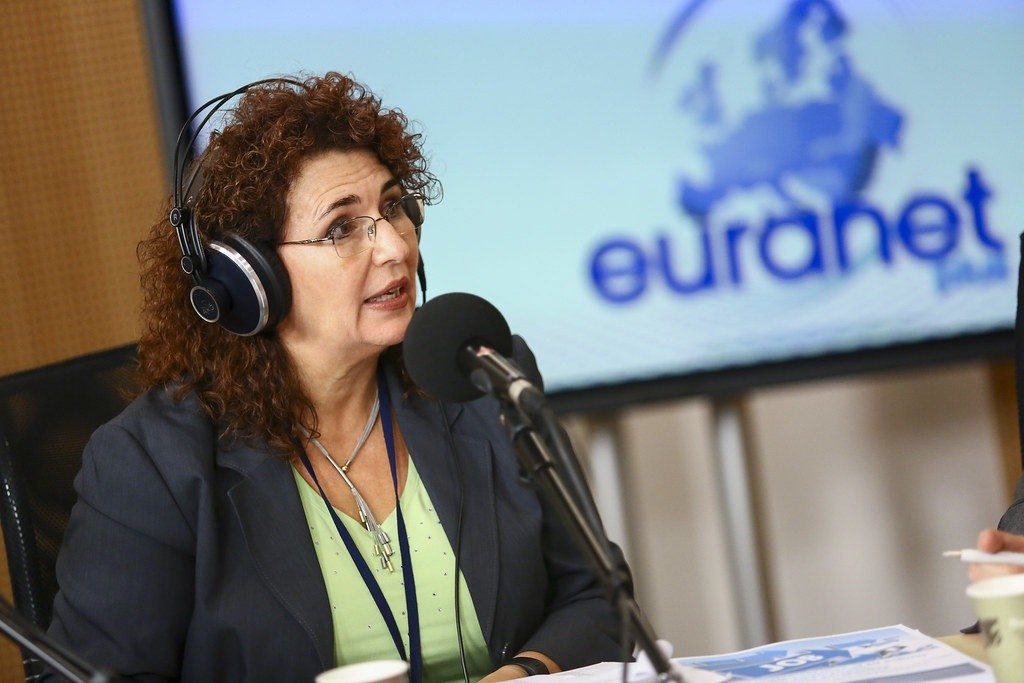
274 192 429 260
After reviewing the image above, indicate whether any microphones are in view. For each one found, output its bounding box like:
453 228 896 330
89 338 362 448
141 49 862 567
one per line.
401 292 547 420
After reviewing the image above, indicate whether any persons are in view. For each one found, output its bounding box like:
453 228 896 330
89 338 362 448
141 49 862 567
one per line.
43 71 639 683
960 471 1024 634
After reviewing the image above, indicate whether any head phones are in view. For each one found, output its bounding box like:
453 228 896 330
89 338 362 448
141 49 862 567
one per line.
169 77 426 338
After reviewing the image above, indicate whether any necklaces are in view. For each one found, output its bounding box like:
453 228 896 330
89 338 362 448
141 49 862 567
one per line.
294 393 395 572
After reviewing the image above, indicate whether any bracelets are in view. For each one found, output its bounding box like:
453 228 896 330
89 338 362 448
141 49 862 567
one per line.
499 642 551 675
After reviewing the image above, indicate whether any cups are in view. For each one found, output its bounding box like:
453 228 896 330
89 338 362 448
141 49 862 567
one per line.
965 574 1024 683
315 660 411 683
